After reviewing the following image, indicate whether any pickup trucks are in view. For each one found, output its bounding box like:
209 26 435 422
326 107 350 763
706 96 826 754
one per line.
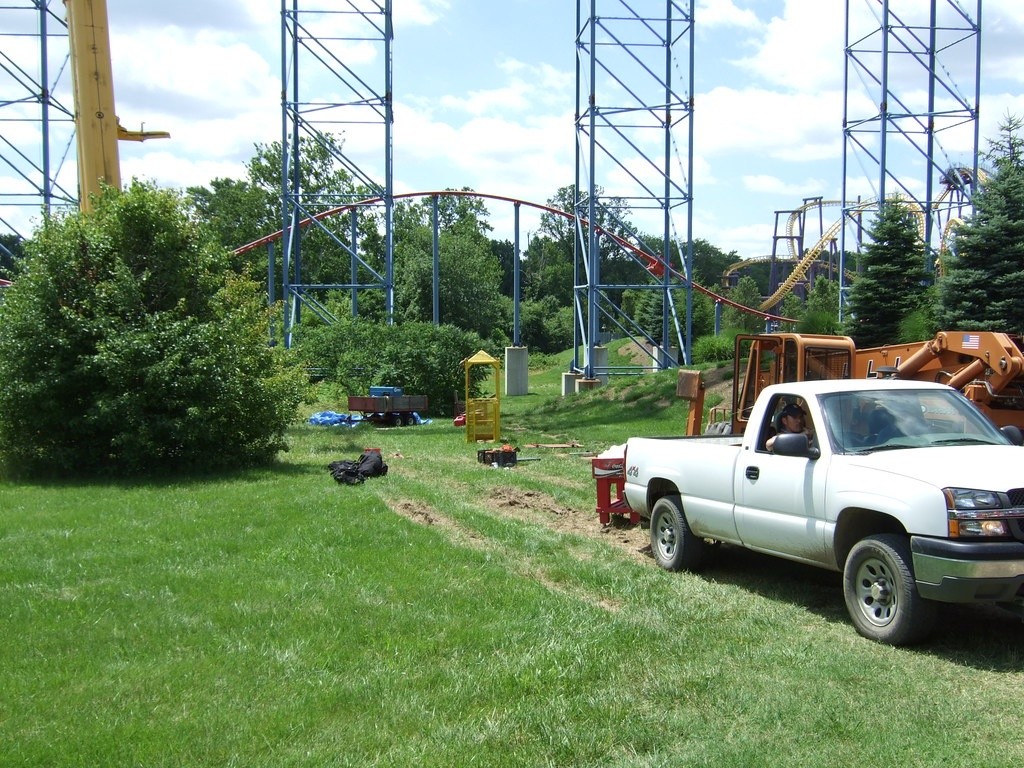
623 380 1024 647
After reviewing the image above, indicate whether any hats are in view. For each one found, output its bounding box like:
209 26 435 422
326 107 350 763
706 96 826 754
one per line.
781 403 807 416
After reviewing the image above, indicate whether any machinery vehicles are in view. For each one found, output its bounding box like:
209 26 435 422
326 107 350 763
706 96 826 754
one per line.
675 329 1024 437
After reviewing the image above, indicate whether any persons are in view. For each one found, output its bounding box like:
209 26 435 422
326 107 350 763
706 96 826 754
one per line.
765 404 814 455
